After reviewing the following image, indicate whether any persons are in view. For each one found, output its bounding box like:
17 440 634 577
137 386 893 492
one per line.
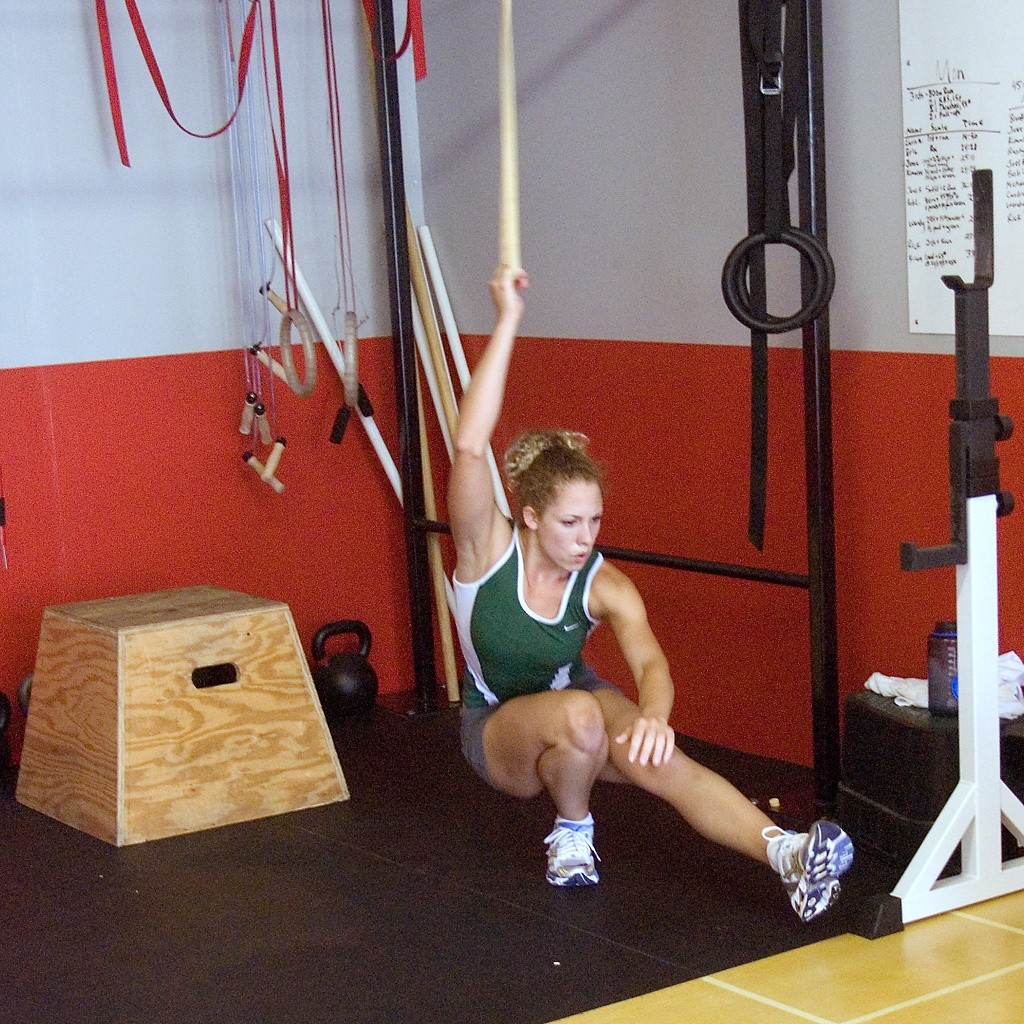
446 261 855 923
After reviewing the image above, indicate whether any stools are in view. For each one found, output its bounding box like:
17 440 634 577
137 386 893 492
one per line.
15 587 351 849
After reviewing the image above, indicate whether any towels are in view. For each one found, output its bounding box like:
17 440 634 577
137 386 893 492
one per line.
862 650 1023 721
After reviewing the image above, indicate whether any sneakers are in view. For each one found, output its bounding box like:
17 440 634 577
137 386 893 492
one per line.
760 820 853 923
543 814 601 887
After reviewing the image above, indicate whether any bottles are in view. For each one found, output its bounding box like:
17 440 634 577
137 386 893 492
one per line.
927 620 958 717
